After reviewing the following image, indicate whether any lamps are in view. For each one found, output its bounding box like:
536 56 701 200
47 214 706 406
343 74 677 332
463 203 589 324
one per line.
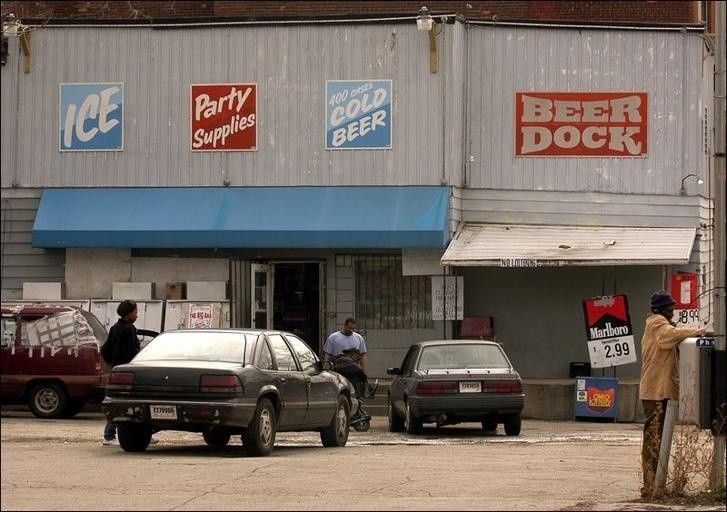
416 4 449 35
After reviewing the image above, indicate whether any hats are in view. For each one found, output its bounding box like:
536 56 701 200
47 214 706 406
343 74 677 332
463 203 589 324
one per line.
117 300 136 315
650 291 675 308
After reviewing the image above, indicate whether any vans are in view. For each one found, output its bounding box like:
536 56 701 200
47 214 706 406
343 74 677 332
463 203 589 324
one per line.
1 304 114 419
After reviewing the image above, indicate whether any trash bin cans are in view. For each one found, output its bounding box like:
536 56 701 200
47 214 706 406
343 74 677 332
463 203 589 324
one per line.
455 315 495 342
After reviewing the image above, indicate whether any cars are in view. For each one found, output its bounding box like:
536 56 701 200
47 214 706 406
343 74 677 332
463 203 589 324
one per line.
387 338 526 437
101 328 359 457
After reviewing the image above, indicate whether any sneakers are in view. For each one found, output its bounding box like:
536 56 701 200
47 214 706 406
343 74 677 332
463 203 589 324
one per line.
150 438 159 444
640 487 671 498
103 439 119 445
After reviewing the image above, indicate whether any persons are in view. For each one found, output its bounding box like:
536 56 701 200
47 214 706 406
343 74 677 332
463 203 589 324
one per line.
323 319 368 369
639 289 713 500
101 300 159 446
330 348 367 386
